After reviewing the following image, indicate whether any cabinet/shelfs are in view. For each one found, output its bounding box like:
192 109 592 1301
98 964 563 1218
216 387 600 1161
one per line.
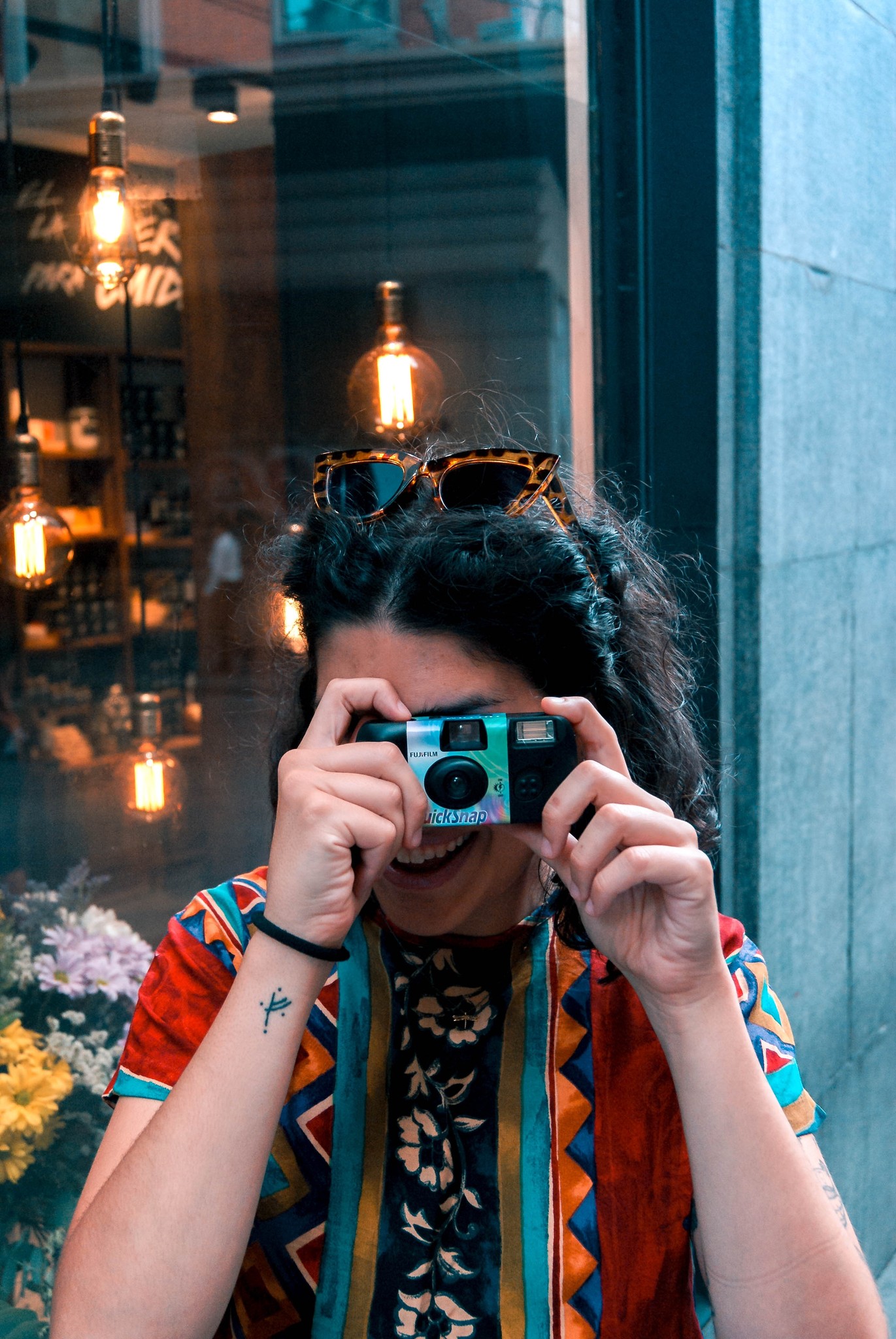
0 339 197 652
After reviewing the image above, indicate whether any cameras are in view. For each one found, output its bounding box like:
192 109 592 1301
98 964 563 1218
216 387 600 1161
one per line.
356 718 577 828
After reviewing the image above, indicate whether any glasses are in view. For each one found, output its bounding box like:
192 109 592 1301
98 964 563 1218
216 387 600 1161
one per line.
311 446 622 603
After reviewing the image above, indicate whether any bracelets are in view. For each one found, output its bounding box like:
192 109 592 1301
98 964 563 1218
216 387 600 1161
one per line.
249 912 351 962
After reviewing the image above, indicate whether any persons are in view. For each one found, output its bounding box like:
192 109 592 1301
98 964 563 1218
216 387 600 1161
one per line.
51 449 889 1339
198 515 245 674
